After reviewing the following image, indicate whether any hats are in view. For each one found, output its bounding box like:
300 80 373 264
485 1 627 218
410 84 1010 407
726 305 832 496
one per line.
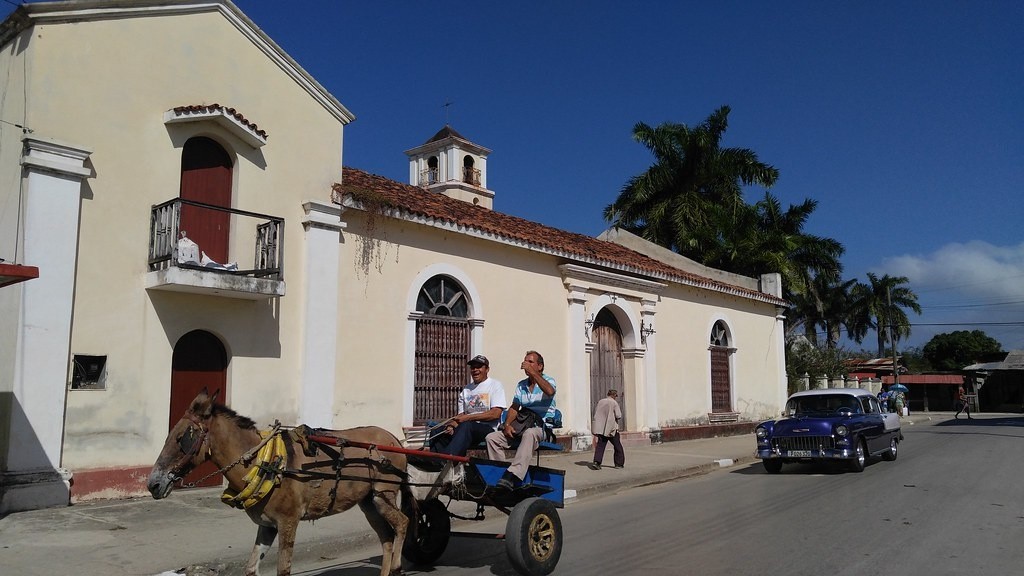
607 390 618 397
467 355 489 366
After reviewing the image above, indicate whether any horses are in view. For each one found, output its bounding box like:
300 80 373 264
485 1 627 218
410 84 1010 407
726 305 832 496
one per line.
147 390 418 576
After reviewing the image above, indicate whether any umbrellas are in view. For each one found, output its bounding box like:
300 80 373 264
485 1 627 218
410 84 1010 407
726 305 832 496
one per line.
890 384 909 395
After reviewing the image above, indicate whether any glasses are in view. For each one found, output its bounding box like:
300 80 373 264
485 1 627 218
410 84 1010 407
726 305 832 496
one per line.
471 364 484 368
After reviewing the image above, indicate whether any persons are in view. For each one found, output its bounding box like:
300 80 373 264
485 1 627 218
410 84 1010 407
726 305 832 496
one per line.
424 355 507 471
888 390 906 417
955 383 973 420
591 389 625 470
838 398 861 414
486 351 557 493
544 409 562 444
877 388 888 413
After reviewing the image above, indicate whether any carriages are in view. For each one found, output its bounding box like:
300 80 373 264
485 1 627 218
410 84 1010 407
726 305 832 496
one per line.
146 386 567 576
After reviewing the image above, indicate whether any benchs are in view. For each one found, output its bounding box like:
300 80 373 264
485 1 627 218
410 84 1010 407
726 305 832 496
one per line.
708 412 740 424
402 426 429 443
427 409 563 451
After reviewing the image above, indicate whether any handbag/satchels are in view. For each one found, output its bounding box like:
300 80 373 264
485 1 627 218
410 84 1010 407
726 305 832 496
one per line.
498 407 556 443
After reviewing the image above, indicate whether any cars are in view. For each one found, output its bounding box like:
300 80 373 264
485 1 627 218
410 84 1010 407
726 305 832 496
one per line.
753 387 904 474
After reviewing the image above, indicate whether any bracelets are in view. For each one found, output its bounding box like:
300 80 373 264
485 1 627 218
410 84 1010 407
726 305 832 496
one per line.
883 398 884 399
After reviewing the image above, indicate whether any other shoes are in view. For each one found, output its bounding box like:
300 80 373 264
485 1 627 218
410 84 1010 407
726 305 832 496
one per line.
496 474 514 492
592 462 601 470
615 464 624 469
968 418 973 419
955 415 958 419
